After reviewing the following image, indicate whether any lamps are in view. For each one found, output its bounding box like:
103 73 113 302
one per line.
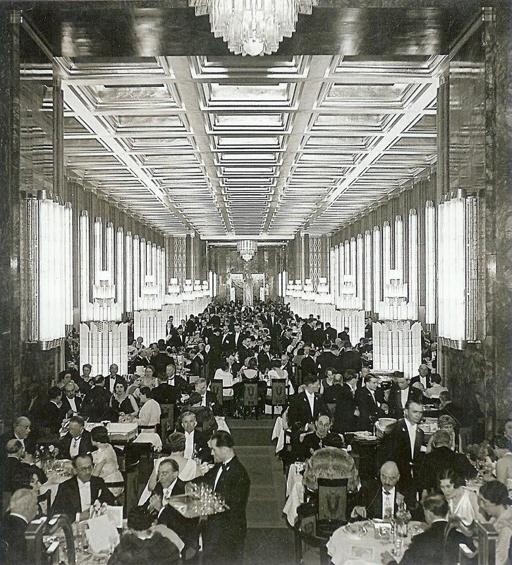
235 240 259 263
182 0 320 58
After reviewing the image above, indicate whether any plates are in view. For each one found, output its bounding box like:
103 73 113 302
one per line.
344 525 367 536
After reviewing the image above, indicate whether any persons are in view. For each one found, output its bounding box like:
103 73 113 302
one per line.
349 460 425 522
51 454 115 524
128 301 366 419
166 411 212 461
295 412 344 460
415 430 478 495
138 433 202 505
381 487 478 563
385 398 424 486
90 426 125 498
141 459 200 539
2 416 33 463
2 488 49 564
108 506 182 564
199 430 250 562
303 433 360 497
281 363 464 451
483 435 511 490
492 419 511 448
2 439 47 490
23 362 217 466
478 481 511 564
52 416 97 460
426 415 463 454
439 467 484 525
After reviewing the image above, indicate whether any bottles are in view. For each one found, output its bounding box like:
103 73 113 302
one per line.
395 501 408 537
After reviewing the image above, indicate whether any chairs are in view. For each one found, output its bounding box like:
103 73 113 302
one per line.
0 301 511 565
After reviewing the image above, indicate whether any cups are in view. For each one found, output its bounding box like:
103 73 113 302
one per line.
184 482 224 517
60 525 91 563
378 526 419 556
